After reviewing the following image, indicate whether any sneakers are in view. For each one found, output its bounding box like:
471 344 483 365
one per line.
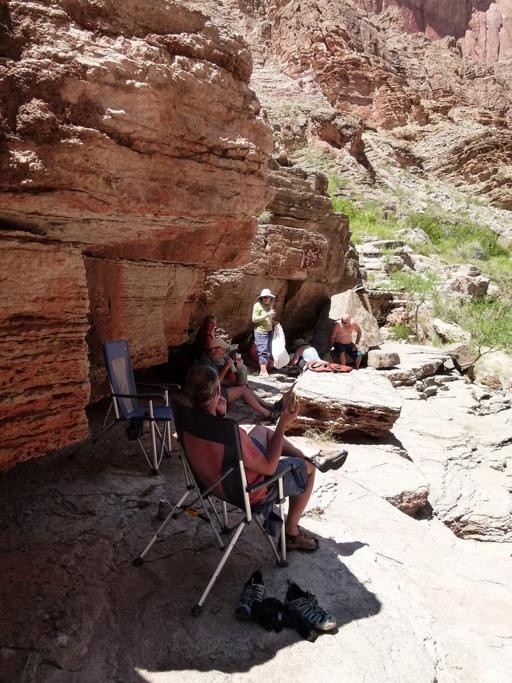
311 449 348 473
259 370 270 379
284 582 337 632
239 569 265 619
277 524 319 553
266 397 284 423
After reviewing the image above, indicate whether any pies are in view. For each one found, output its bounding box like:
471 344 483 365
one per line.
281 391 296 413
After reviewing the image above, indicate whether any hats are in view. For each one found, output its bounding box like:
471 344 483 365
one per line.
256 289 276 300
290 338 309 349
208 337 233 350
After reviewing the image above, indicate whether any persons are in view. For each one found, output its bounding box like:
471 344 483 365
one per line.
328 313 363 369
188 315 218 358
251 288 278 378
199 337 278 420
287 327 353 372
185 367 348 552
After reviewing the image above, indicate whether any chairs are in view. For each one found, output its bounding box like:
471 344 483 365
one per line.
69 339 181 478
134 398 307 619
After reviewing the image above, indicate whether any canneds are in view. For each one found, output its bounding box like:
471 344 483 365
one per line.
157 499 169 520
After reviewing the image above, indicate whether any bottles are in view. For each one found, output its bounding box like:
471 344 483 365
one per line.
225 355 238 374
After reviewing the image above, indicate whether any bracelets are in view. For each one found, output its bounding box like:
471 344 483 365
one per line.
354 343 358 348
